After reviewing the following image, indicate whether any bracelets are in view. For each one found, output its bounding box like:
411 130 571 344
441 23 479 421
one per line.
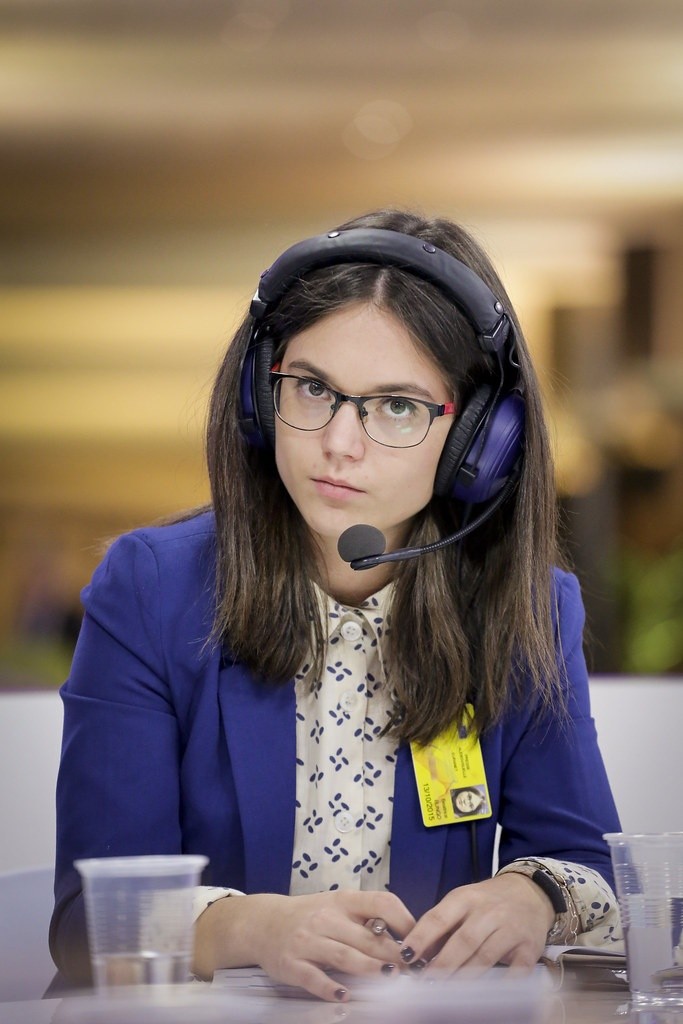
544 870 580 947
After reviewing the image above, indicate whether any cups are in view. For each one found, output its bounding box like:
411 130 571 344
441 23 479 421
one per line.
603 832 683 1010
74 856 209 1006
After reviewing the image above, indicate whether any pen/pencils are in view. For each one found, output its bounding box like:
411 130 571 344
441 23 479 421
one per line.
370 918 387 936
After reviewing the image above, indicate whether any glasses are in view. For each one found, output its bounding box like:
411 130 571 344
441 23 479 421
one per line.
267 362 456 448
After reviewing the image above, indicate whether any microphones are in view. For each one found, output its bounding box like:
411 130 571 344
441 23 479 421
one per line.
338 458 524 571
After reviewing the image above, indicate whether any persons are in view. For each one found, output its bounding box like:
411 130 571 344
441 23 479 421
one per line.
41 209 643 1003
449 787 486 818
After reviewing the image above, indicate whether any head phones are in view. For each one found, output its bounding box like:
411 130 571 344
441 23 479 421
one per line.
238 226 528 507
